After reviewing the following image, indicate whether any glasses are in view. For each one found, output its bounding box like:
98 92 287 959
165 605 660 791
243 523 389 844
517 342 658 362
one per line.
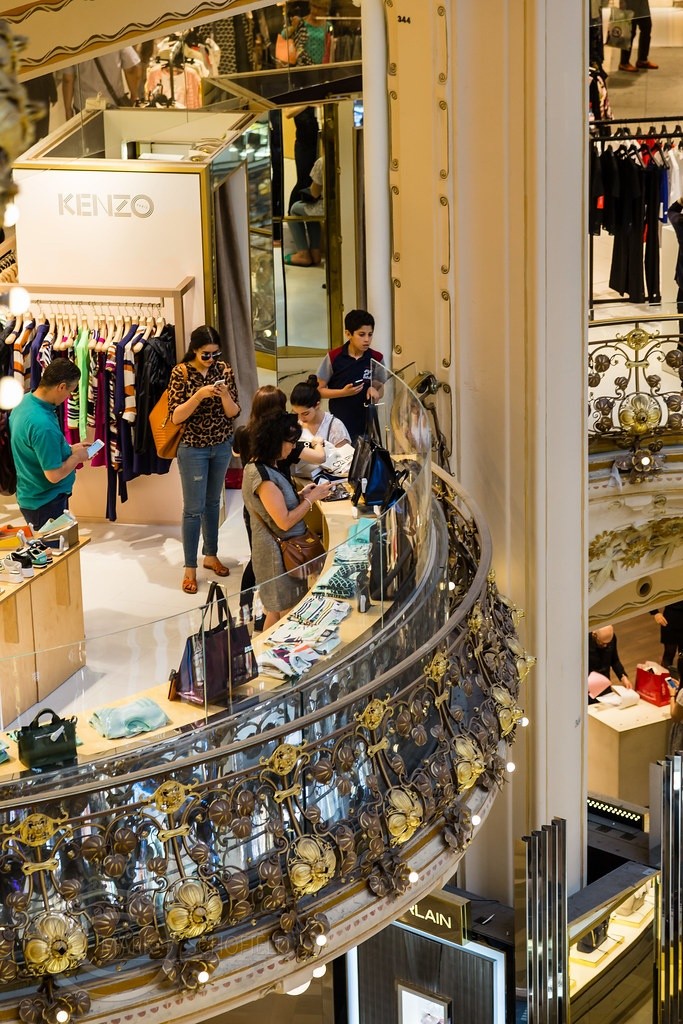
200 350 222 360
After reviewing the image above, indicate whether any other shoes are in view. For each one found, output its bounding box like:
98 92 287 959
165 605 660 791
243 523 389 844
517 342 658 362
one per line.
0 524 33 551
36 514 74 537
635 60 658 69
619 62 639 72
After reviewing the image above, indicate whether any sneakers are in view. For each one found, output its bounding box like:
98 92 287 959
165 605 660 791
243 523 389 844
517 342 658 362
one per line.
24 539 54 564
14 546 47 568
2 551 35 578
0 558 23 583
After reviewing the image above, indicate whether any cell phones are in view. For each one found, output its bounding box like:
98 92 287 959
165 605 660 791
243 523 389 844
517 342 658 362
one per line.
213 378 225 387
354 379 363 387
87 439 105 459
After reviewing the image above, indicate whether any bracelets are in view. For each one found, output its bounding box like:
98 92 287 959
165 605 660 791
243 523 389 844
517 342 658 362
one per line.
303 497 313 512
194 393 201 403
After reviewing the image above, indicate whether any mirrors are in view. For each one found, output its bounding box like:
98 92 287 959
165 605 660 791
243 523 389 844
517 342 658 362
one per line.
275 104 332 357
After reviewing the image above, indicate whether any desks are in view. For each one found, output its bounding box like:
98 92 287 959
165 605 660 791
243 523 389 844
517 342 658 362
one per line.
0 537 94 731
586 690 681 810
0 476 417 783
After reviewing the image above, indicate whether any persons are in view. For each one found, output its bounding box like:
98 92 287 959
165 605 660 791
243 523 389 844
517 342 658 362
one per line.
232 384 325 635
281 0 334 67
317 309 386 445
588 624 632 705
284 103 327 266
650 600 683 722
8 358 99 533
289 374 351 448
618 0 659 71
166 325 241 594
10 47 142 146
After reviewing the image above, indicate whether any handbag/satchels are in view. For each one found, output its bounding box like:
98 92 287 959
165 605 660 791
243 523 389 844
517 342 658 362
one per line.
275 34 297 65
149 362 188 460
605 7 634 49
176 579 260 707
636 661 672 707
275 524 323 579
367 468 417 601
347 403 399 505
13 708 78 771
298 189 321 204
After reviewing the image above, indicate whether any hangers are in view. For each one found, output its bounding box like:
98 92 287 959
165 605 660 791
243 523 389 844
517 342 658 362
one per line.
596 116 683 167
5 299 167 355
151 32 212 79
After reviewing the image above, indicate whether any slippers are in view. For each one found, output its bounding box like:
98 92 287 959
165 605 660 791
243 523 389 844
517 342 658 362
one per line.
203 556 230 577
284 252 314 268
182 570 198 594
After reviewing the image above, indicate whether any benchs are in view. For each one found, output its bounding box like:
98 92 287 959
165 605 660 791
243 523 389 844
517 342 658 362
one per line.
283 215 322 251
